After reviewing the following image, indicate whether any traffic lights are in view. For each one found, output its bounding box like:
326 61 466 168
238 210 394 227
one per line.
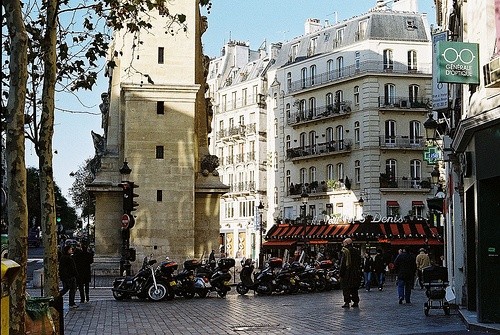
57 211 62 223
122 180 140 213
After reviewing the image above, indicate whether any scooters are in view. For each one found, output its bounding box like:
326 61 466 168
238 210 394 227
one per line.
137 250 236 298
236 248 342 296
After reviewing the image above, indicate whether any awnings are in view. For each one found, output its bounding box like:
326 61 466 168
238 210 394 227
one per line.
262 239 297 248
390 239 444 245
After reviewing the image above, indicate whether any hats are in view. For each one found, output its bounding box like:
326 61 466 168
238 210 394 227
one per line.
419 248 426 251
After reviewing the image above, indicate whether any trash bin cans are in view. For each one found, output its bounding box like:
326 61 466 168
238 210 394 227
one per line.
32 269 45 286
26 297 55 335
0 258 20 335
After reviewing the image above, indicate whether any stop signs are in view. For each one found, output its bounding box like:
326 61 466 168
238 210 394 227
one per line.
121 214 131 230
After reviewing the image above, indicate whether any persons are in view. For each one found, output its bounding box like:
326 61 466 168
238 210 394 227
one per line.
362 252 375 291
59 245 79 309
290 248 341 264
209 249 217 268
74 244 94 303
339 238 362 308
375 249 387 291
394 247 417 303
416 248 431 289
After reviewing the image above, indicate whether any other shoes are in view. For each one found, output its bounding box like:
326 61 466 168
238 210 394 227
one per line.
406 300 411 303
379 288 382 291
366 288 370 291
342 303 349 307
351 302 358 307
399 297 403 304
80 301 84 303
87 299 89 302
69 305 78 308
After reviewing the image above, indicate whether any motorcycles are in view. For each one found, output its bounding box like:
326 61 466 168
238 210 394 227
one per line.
110 245 168 302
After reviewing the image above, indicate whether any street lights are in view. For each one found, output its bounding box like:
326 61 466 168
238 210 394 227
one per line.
256 201 266 270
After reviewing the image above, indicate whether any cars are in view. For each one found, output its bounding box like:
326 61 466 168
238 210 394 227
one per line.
64 238 78 246
29 237 41 248
